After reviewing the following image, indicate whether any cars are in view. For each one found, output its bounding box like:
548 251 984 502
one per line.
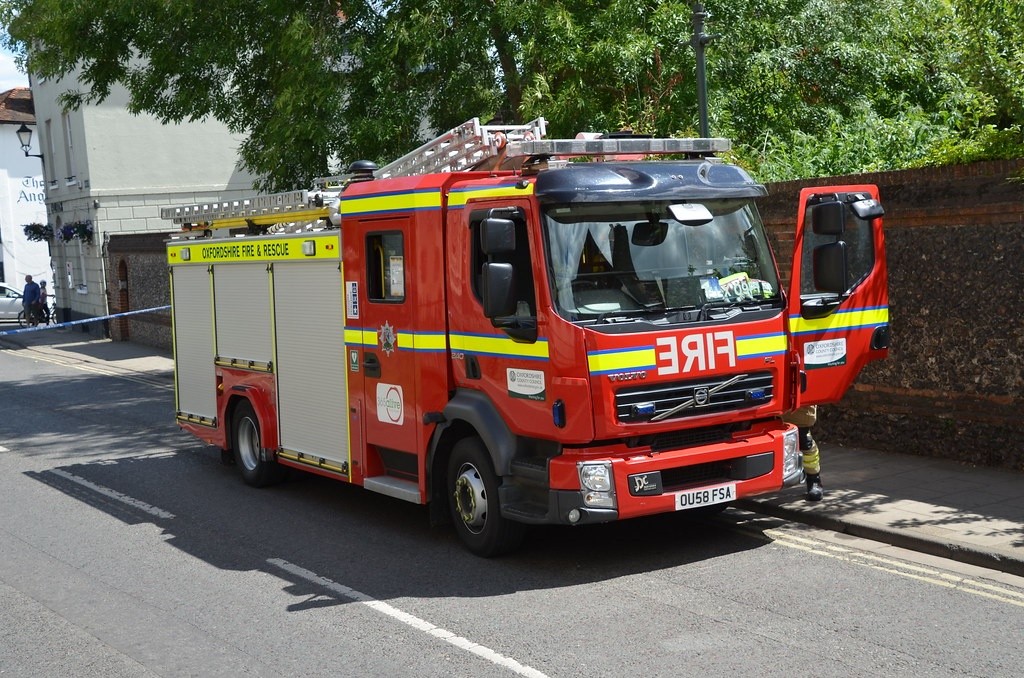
0 281 38 322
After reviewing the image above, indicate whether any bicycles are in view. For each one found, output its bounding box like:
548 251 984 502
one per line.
17 293 57 329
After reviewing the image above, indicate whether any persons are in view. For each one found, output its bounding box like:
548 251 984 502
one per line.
784 405 824 501
22 275 50 327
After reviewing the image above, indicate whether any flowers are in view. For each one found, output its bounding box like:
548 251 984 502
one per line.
23 222 54 243
54 221 93 245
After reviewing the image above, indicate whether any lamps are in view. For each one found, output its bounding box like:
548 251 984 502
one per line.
16 124 45 167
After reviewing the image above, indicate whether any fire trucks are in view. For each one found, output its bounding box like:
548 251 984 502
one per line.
155 116 890 559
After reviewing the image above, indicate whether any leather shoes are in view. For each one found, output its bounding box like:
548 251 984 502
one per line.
806 474 823 501
